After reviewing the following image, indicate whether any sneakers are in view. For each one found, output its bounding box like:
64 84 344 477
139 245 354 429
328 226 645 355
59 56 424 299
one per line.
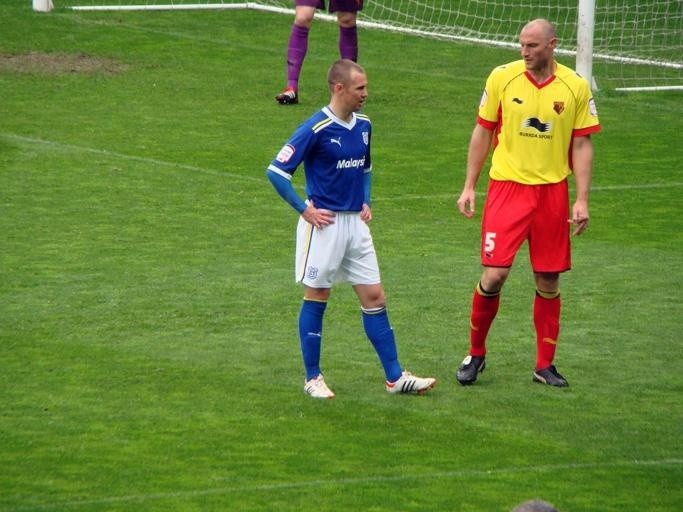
385 370 436 394
456 353 485 385
275 88 299 104
304 372 335 399
532 364 569 387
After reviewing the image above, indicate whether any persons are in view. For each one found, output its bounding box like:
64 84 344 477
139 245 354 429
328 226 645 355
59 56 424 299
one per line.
263 58 435 400
453 18 601 389
274 1 363 105
